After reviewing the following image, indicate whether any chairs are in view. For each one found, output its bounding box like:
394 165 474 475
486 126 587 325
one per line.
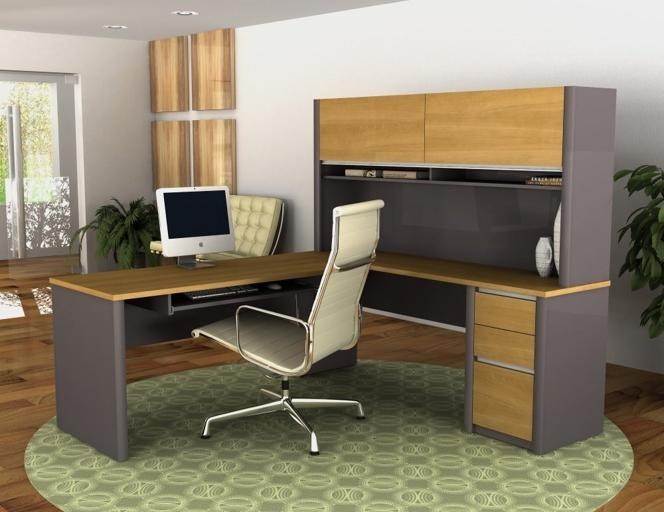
196 195 285 261
191 199 385 455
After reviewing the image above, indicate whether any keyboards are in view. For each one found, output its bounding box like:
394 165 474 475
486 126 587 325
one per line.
185 285 258 300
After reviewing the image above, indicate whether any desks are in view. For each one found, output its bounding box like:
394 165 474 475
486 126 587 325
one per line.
49 251 331 461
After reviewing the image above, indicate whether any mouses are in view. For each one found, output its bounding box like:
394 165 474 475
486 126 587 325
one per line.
268 284 282 290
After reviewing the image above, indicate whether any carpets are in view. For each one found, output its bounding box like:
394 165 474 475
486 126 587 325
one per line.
23 362 636 512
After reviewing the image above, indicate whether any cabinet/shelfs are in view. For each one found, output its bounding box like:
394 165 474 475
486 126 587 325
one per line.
359 267 469 334
313 87 618 453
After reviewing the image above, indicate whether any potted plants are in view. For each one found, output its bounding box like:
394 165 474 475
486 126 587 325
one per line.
68 196 162 271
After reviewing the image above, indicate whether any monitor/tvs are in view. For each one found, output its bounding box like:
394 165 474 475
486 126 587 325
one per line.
156 185 236 270
